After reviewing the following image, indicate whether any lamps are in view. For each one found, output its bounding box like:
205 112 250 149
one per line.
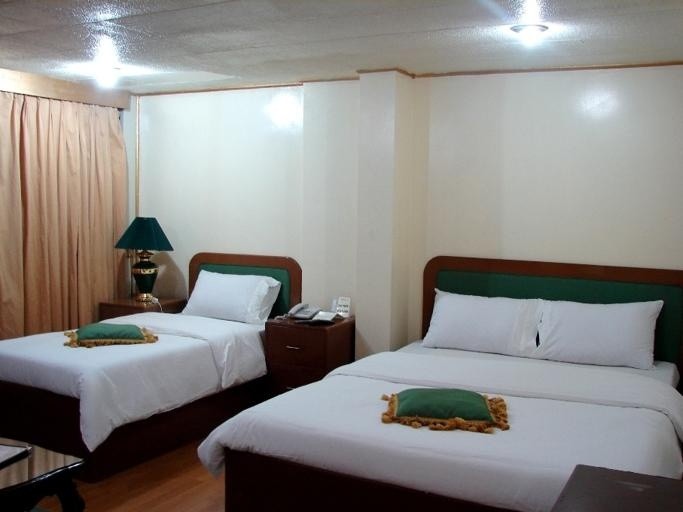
113 215 173 303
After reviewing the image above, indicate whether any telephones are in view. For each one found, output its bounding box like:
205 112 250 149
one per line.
287 303 321 320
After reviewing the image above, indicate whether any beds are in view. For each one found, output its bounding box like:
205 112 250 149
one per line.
196 254 683 512
2 250 304 486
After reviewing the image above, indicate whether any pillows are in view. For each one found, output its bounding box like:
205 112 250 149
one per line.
532 300 665 371
421 287 543 359
63 322 158 350
182 270 281 328
377 387 510 434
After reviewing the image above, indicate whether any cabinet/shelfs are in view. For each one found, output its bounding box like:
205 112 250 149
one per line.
94 297 186 326
261 317 355 397
